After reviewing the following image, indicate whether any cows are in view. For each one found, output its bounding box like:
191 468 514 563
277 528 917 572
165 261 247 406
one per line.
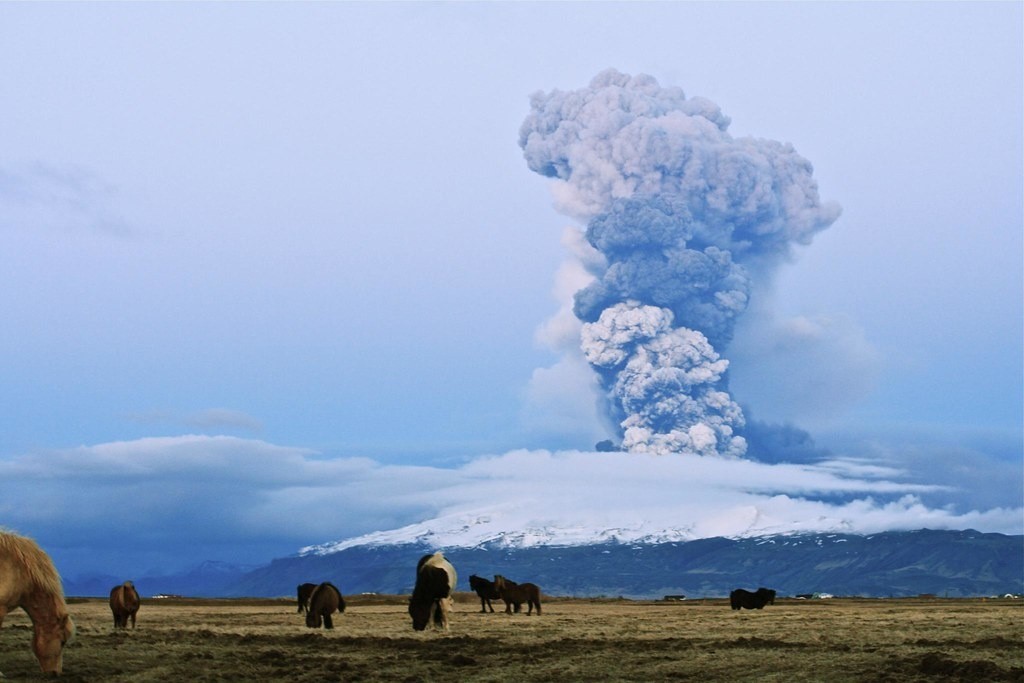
295 581 347 630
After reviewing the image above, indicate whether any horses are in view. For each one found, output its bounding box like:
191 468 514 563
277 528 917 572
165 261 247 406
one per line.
730 587 777 611
409 551 458 634
0 526 76 683
469 574 543 617
110 581 140 629
